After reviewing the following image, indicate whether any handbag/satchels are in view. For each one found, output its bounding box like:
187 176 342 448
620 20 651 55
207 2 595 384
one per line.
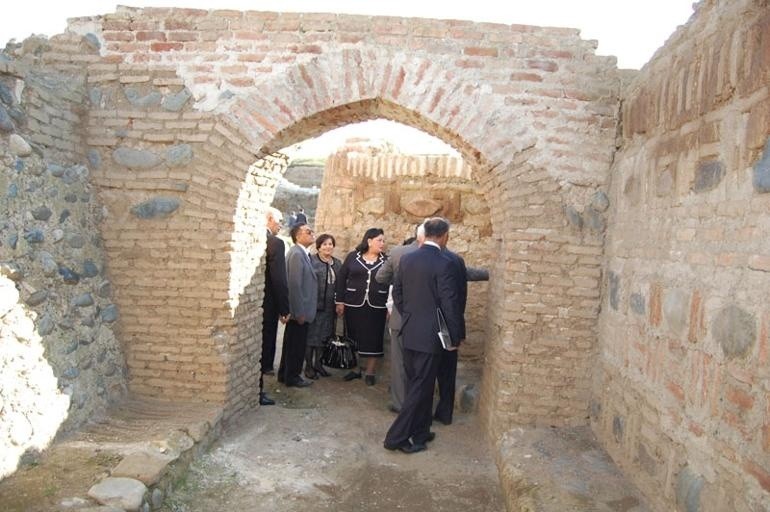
322 334 357 370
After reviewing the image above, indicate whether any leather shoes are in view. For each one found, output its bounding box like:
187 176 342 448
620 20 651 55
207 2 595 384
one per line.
414 433 435 445
344 371 361 381
287 380 310 387
262 397 275 405
366 374 375 386
384 439 417 453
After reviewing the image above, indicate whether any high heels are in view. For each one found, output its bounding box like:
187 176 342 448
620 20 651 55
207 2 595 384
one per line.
305 367 330 380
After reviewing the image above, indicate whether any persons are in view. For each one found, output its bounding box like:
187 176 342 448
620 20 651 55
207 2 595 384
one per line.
278 213 318 387
259 207 292 405
304 233 342 379
334 228 389 385
375 217 489 452
